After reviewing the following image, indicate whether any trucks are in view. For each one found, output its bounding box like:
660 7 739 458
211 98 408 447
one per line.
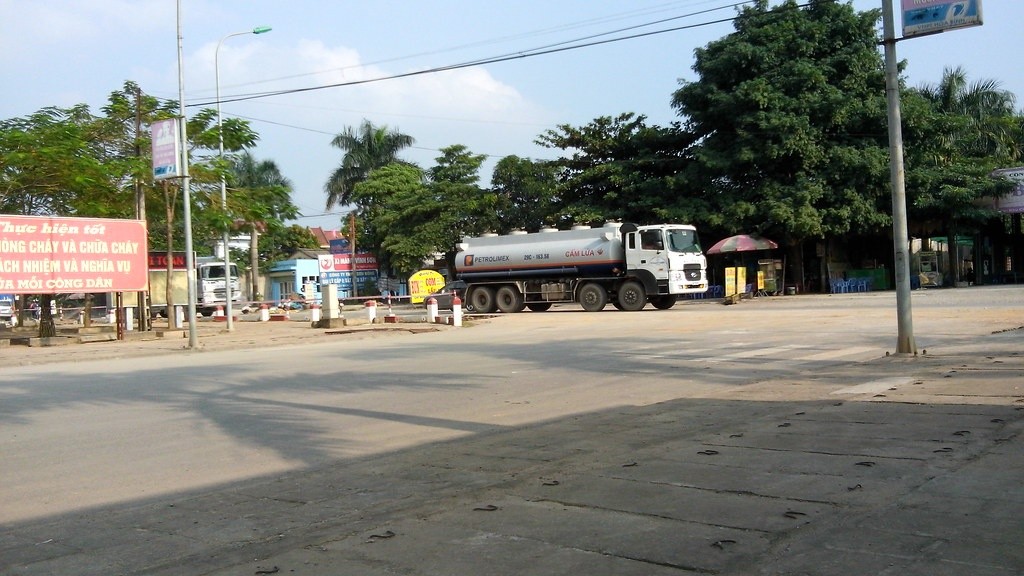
191 256 241 317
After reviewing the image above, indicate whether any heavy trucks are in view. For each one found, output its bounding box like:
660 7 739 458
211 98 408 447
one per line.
450 217 708 313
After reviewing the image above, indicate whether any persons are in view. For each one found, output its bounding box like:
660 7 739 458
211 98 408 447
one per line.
59 304 64 320
30 300 39 319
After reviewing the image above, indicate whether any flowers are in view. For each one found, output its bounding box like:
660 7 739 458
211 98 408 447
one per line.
269 309 286 314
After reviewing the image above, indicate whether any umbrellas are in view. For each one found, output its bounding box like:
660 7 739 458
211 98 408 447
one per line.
707 234 778 254
65 293 95 301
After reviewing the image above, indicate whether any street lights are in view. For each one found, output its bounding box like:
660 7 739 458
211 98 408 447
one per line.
215 25 273 332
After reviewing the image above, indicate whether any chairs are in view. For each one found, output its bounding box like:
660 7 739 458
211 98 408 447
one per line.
911 274 919 289
828 277 871 294
685 284 753 299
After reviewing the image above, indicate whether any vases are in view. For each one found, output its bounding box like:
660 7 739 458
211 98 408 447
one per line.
384 317 398 323
270 315 286 321
435 317 453 324
213 316 237 321
